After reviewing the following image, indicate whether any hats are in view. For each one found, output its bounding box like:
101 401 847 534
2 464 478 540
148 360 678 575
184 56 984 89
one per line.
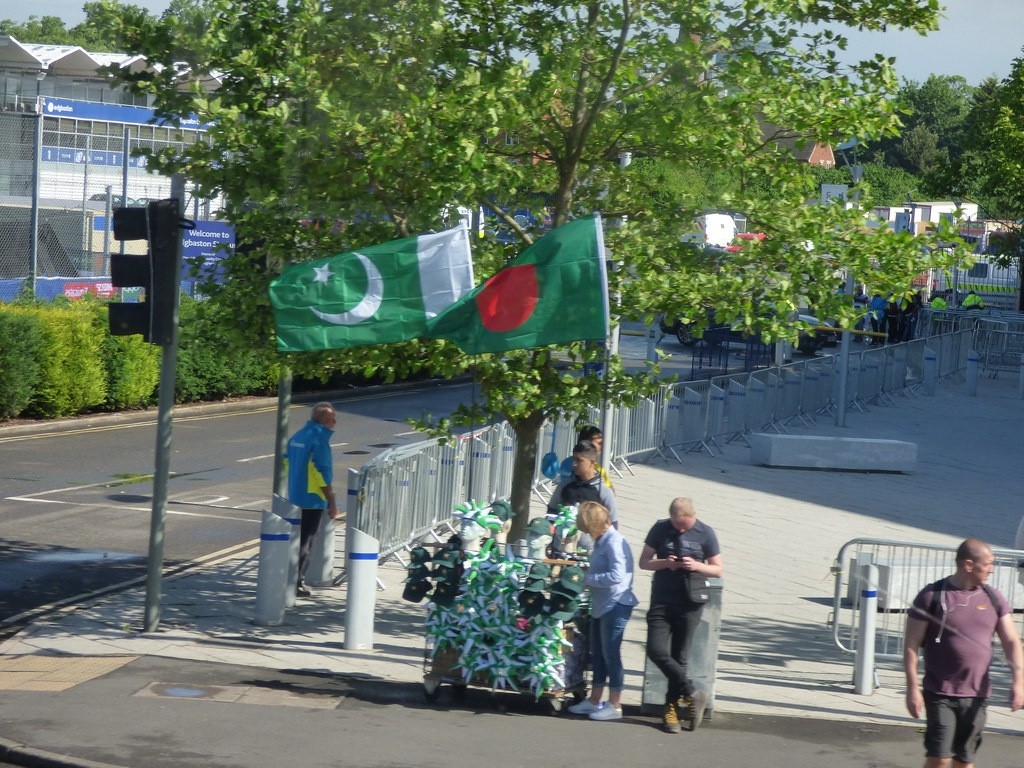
492 500 513 522
523 518 555 536
516 563 584 621
401 546 464 607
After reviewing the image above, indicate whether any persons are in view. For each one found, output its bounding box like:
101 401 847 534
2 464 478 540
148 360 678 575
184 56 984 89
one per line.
520 517 554 560
545 424 618 533
286 402 337 596
568 501 639 720
459 508 490 554
639 497 722 733
834 282 984 346
904 538 1024 768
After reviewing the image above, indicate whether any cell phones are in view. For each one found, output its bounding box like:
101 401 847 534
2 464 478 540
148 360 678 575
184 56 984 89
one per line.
675 558 684 563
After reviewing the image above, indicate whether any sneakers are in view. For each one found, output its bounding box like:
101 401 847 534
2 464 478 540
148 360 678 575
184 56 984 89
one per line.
687 690 709 731
589 700 623 720
568 700 604 715
663 703 681 733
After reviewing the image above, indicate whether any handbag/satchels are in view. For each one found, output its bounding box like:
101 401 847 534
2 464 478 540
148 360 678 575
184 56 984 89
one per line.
683 571 710 603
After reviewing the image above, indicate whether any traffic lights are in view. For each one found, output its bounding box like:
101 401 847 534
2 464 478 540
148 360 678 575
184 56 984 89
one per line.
108 203 156 345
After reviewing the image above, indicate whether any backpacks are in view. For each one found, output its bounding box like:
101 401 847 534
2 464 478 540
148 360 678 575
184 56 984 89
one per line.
565 478 600 507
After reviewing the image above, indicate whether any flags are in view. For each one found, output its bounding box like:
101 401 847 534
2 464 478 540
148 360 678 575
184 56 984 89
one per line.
425 212 610 355
271 219 475 352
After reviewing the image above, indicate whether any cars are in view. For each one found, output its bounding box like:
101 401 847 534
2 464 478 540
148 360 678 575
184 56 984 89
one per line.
659 298 839 354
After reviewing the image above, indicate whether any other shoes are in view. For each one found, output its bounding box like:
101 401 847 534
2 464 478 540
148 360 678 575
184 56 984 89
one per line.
296 579 310 597
